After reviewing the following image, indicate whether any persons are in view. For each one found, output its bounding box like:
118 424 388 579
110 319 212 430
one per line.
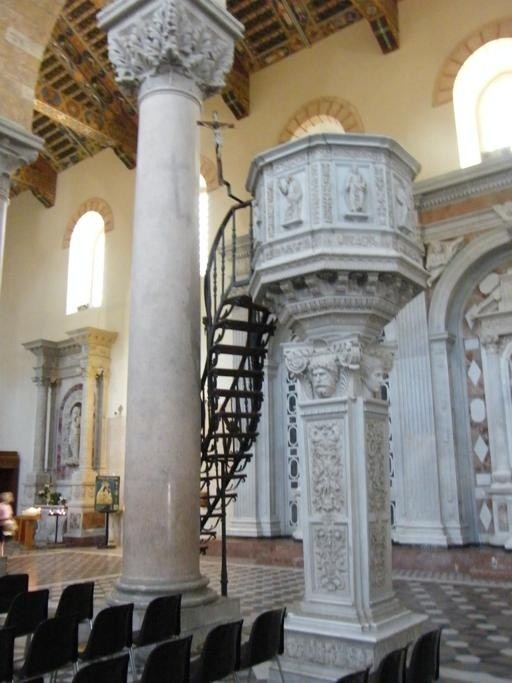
67 406 80 460
360 354 384 393
0 491 17 558
308 353 338 398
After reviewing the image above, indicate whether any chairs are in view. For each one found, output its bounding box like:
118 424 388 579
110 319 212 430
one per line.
335 627 446 683
0 573 287 683
